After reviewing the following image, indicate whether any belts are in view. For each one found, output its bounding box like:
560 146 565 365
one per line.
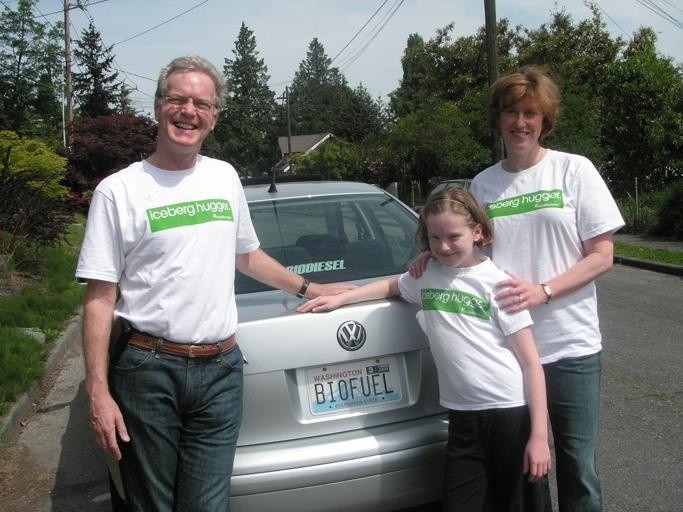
125 329 237 358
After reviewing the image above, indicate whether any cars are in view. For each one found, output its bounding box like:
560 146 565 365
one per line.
228 164 452 512
430 179 475 197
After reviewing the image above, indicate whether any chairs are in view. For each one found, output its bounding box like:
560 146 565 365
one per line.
267 234 394 282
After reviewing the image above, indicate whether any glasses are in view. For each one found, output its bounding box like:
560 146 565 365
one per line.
164 94 218 111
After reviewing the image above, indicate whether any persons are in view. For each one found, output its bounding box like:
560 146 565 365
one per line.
407 63 626 510
74 57 365 511
294 186 553 511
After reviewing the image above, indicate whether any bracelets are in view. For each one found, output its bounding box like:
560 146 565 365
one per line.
295 279 309 298
541 283 551 304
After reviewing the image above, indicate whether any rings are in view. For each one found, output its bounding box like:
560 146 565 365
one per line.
518 294 521 302
